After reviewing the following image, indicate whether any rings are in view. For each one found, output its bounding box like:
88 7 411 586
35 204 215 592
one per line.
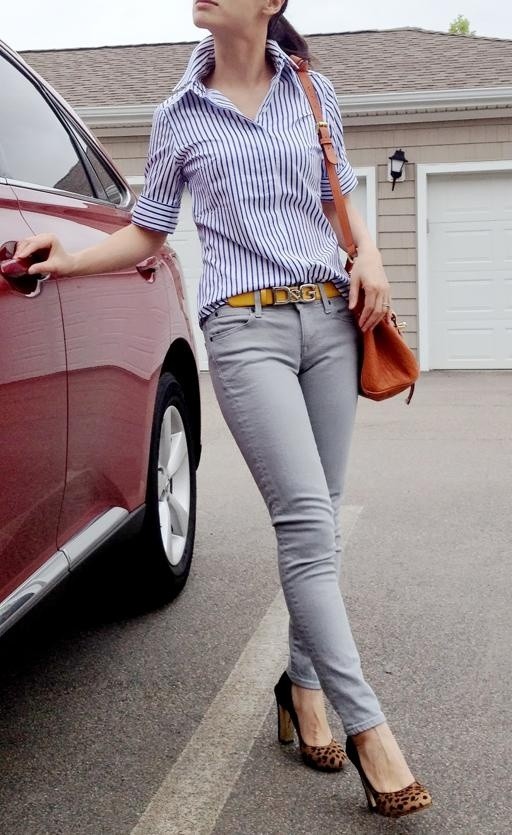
382 303 389 308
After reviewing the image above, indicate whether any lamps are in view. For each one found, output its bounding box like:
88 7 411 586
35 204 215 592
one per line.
386 148 409 192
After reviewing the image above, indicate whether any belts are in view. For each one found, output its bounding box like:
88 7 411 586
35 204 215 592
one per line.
226 283 339 307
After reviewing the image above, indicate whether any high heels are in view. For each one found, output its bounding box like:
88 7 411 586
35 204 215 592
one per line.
273 672 350 773
345 736 432 817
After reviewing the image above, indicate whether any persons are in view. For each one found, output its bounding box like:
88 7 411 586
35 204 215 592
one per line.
8 0 432 818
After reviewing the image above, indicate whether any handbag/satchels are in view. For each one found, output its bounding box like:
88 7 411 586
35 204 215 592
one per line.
346 253 419 403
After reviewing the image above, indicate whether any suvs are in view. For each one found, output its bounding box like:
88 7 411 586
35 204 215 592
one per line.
0 42 199 638
